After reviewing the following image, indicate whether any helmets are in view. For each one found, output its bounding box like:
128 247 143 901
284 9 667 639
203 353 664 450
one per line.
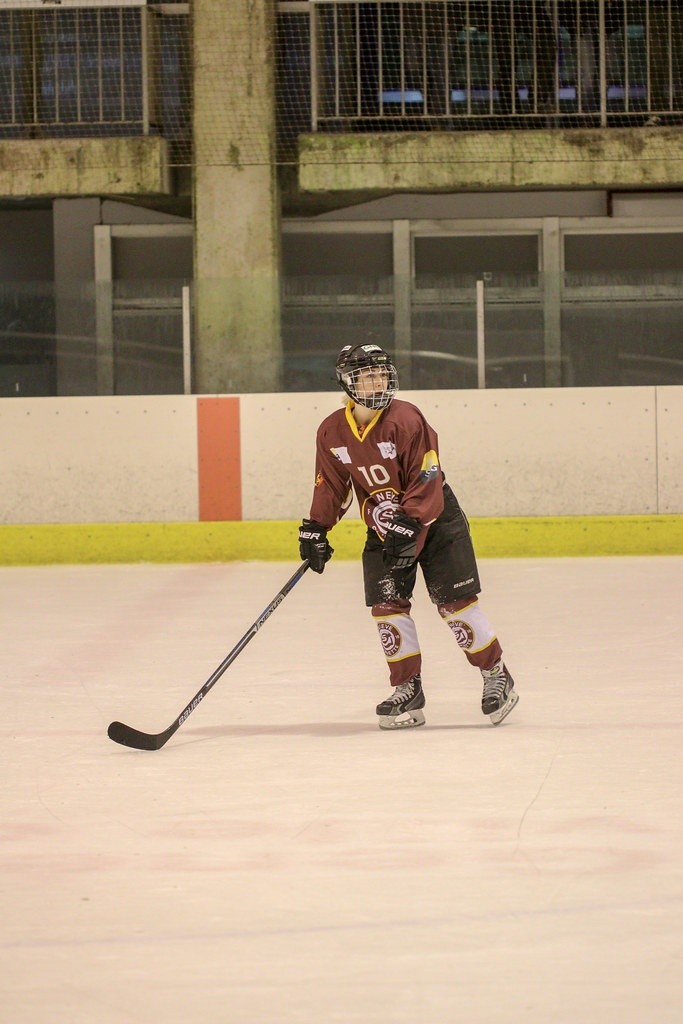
335 343 396 410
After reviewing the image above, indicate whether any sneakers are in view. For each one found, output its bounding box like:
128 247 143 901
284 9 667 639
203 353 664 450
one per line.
376 675 427 730
481 661 519 724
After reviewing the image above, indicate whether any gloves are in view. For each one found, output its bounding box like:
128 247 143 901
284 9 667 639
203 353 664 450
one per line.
382 516 421 570
298 519 334 574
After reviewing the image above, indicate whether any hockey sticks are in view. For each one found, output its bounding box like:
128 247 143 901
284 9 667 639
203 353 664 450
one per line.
105 559 312 752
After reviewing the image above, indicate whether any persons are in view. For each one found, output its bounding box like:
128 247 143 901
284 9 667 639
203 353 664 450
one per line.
298 343 519 730
